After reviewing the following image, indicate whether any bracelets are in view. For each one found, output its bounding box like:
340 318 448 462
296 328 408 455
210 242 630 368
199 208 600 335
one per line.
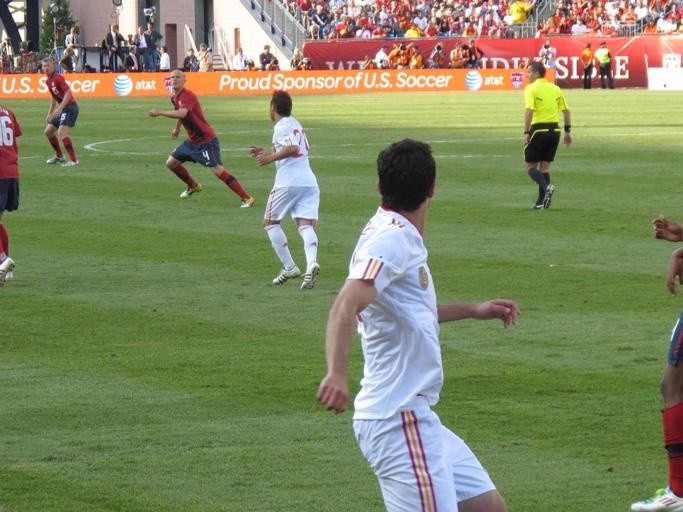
524 131 531 135
564 124 572 132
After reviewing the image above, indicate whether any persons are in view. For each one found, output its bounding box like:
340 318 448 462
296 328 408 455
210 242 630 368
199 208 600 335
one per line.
594 41 614 89
59 25 97 73
0 103 22 284
628 215 683 511
184 44 215 73
1 38 55 73
314 137 521 512
147 69 254 209
580 42 594 89
281 1 683 71
102 22 171 72
233 44 279 72
35 58 80 167
248 89 320 290
521 60 573 211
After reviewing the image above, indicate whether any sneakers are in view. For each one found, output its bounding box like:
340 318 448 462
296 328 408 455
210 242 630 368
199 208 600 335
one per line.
543 184 555 208
301 262 319 290
239 195 255 208
63 160 78 168
630 487 682 512
0 255 16 283
47 155 66 163
272 265 300 284
179 183 201 198
